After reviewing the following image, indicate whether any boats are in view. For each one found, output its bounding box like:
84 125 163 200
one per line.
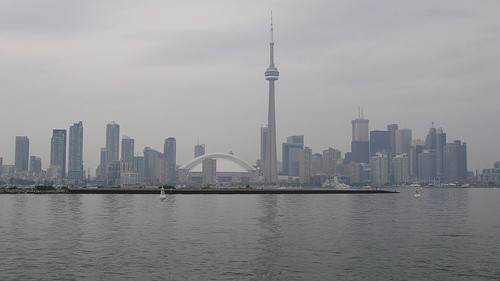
414 189 420 197
336 184 351 189
160 186 167 199
